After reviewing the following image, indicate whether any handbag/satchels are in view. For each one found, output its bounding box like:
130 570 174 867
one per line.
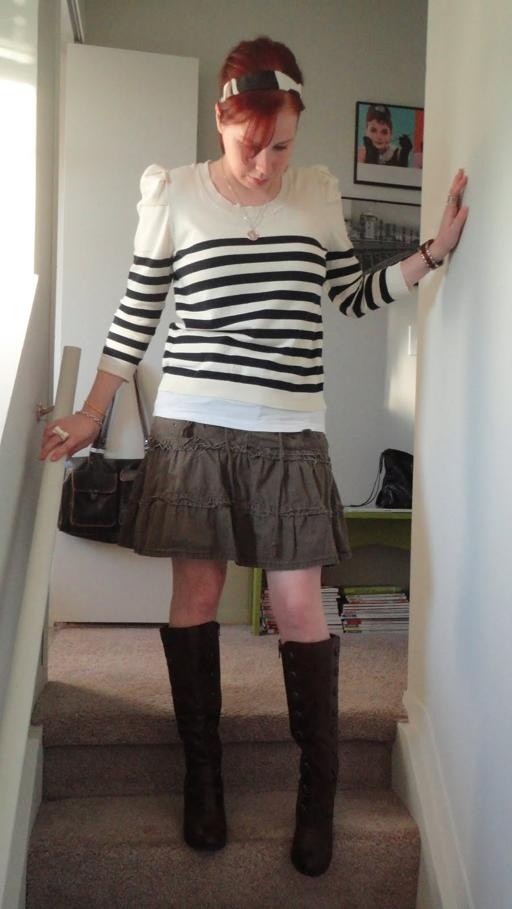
375 447 413 509
59 454 145 544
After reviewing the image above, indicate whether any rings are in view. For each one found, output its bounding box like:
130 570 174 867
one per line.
52 425 70 442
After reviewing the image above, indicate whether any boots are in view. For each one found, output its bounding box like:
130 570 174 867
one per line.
159 620 227 851
274 635 337 877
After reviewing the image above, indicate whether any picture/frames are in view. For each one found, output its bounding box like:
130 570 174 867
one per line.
354 100 425 191
341 195 421 287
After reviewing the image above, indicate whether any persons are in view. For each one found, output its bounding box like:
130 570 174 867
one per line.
356 106 414 167
39 36 469 878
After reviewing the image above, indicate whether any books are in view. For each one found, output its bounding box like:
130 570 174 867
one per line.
263 584 408 635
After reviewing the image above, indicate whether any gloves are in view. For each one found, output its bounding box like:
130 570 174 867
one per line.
363 136 377 163
398 134 412 167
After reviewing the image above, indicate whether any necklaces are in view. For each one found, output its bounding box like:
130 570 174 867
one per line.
221 157 275 240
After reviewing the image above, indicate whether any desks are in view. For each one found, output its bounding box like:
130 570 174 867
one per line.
249 505 413 637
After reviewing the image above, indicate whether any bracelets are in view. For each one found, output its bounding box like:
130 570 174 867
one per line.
85 400 106 417
418 239 444 271
74 409 104 431
